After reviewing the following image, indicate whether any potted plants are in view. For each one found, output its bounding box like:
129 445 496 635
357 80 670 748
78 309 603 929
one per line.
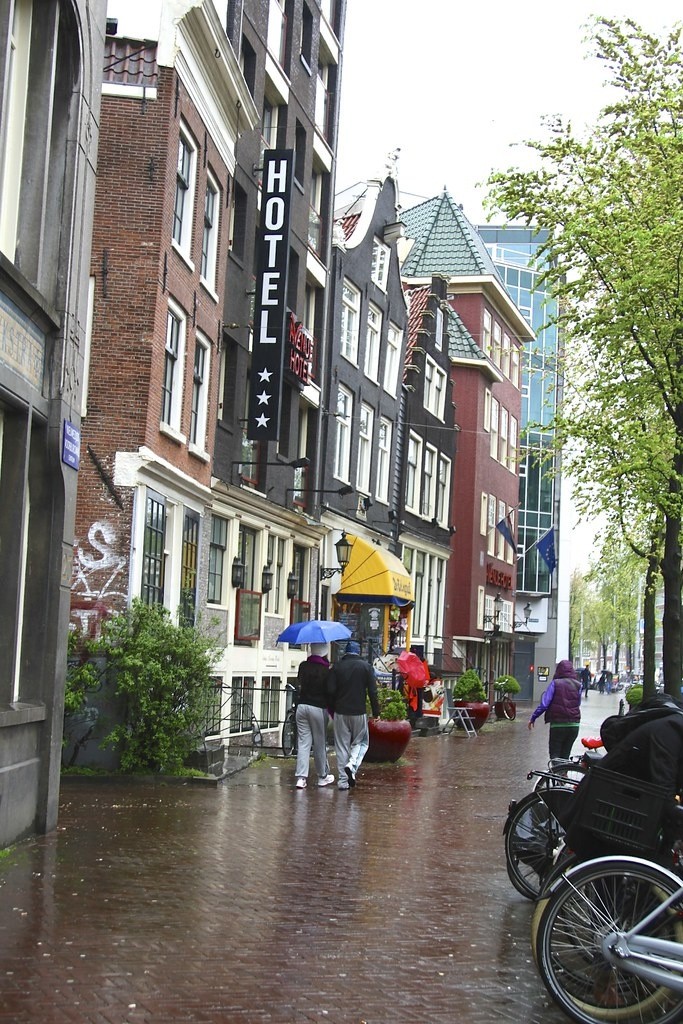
361 688 413 764
494 674 521 720
452 668 491 731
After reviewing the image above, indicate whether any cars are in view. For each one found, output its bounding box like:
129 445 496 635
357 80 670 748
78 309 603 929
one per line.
577 667 664 693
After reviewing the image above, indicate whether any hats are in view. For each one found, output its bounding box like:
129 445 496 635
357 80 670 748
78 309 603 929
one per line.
311 642 328 657
346 641 361 655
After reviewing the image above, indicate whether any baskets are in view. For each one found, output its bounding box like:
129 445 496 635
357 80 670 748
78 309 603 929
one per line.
575 762 670 855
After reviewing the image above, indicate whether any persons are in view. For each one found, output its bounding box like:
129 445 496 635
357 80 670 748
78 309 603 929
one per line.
579 664 592 698
332 641 381 790
597 672 612 695
295 643 335 789
527 660 583 787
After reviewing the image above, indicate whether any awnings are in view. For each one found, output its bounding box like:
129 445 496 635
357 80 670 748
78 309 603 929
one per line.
333 531 416 610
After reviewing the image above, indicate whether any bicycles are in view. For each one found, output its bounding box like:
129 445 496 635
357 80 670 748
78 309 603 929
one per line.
502 737 683 1024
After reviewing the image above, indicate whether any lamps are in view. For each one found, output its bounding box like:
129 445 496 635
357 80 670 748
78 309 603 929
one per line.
335 530 355 572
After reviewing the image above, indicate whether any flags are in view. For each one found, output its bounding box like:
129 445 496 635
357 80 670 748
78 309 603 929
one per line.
496 513 518 553
535 526 556 574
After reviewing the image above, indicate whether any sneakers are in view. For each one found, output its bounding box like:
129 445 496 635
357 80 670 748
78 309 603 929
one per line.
345 764 356 788
295 777 308 788
318 774 335 786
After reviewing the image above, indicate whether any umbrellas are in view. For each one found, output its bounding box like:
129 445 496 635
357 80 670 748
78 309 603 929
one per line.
398 650 426 689
275 618 354 644
600 670 612 675
574 667 586 671
